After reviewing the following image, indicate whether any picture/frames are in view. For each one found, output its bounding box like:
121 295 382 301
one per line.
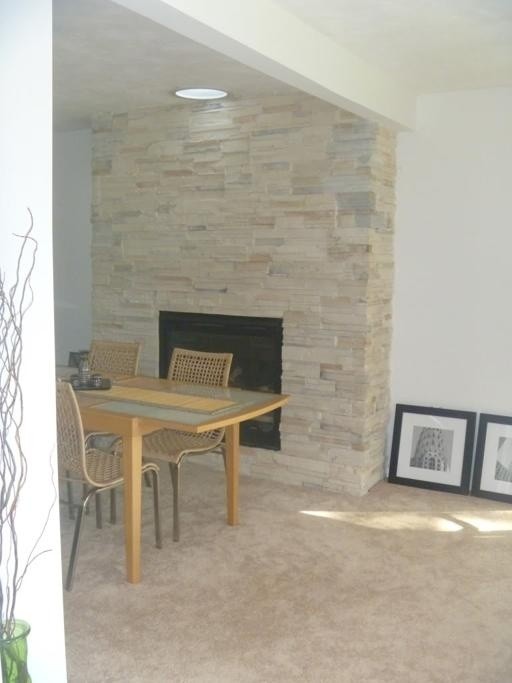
473 414 511 503
385 403 475 494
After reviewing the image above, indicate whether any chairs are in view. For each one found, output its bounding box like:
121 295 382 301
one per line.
56 377 161 591
85 339 148 527
108 347 233 543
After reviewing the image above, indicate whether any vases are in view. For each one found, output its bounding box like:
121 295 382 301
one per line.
2 620 30 681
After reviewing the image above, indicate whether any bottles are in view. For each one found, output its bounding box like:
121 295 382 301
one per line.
79 354 90 386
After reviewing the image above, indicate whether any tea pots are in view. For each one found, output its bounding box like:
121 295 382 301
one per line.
85 374 105 388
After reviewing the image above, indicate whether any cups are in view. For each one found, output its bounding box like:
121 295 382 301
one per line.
70 374 80 388
